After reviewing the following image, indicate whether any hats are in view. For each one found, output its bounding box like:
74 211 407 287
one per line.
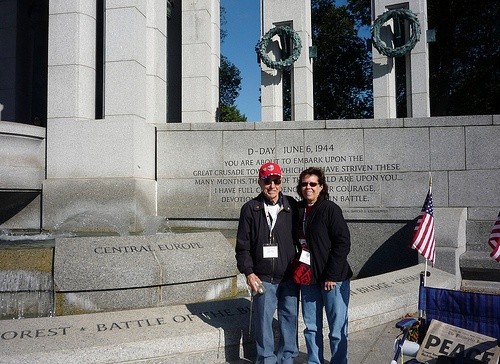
259 162 282 179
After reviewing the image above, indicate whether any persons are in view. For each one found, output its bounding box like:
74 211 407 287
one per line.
235 162 302 364
294 167 354 364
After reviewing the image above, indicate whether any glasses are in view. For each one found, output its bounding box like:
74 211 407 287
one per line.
264 178 281 185
301 182 319 187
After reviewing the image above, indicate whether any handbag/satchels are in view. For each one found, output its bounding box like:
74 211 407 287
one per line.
293 243 312 285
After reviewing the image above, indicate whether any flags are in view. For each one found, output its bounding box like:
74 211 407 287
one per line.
488 211 500 263
408 186 436 269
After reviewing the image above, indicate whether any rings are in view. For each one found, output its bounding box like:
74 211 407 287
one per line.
248 283 251 285
